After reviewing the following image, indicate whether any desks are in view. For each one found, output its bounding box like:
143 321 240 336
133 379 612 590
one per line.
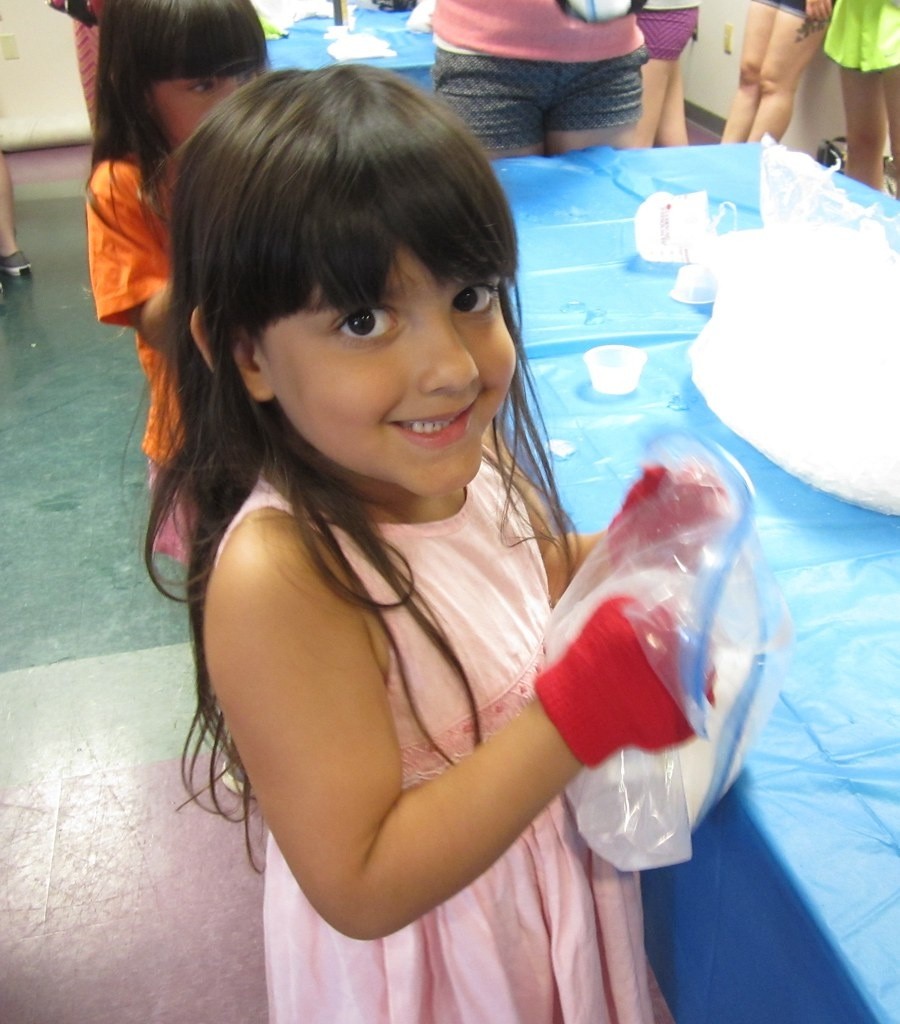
265 6 435 95
480 140 900 1024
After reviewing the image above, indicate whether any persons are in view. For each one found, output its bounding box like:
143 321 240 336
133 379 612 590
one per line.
632 0 697 149
89 0 276 554
48 0 111 139
823 0 900 202
720 0 836 145
143 66 724 1024
1 148 31 303
432 0 642 161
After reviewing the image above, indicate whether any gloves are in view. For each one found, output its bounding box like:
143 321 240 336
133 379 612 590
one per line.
609 463 728 576
535 595 719 772
